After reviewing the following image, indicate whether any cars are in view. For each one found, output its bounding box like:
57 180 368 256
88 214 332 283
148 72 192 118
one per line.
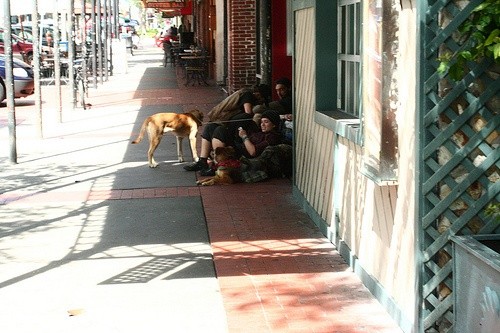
11 23 54 47
0 54 35 104
0 28 69 76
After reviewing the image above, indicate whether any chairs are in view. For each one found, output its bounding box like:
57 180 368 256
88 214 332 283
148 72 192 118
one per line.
164 47 176 68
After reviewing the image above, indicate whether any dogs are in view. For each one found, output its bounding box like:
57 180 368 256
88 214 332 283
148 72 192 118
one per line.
131 109 204 168
195 144 293 186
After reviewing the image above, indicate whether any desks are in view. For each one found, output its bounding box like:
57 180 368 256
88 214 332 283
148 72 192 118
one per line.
178 53 201 77
182 55 210 84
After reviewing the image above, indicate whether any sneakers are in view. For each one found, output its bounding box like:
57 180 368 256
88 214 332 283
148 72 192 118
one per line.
183 160 208 171
198 168 215 175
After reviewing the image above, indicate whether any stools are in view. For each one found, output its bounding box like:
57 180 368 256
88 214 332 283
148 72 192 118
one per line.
185 67 206 87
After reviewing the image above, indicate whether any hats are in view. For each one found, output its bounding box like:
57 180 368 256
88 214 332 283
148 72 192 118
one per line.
261 110 280 128
256 84 272 107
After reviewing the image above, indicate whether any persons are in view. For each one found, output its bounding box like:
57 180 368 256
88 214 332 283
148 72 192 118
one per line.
183 110 293 176
207 78 293 128
160 24 179 49
